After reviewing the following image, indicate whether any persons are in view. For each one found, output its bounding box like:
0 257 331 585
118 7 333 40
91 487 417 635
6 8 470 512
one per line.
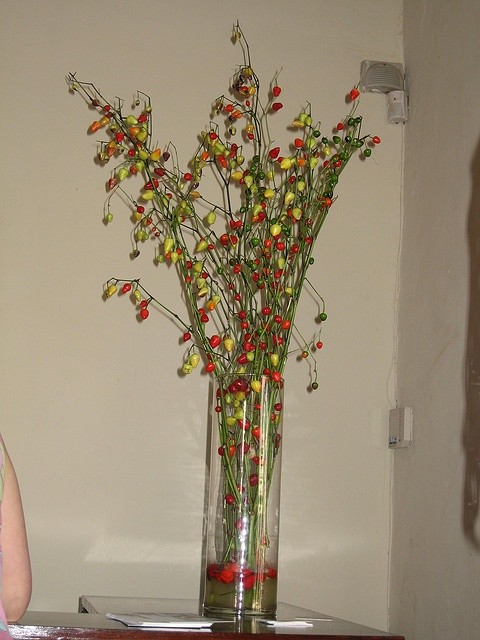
0 432 31 638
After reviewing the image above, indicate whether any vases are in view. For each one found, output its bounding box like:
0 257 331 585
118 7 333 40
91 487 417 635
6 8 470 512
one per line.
199 372 289 621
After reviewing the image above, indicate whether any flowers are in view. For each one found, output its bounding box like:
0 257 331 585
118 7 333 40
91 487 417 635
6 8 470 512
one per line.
62 19 381 611
